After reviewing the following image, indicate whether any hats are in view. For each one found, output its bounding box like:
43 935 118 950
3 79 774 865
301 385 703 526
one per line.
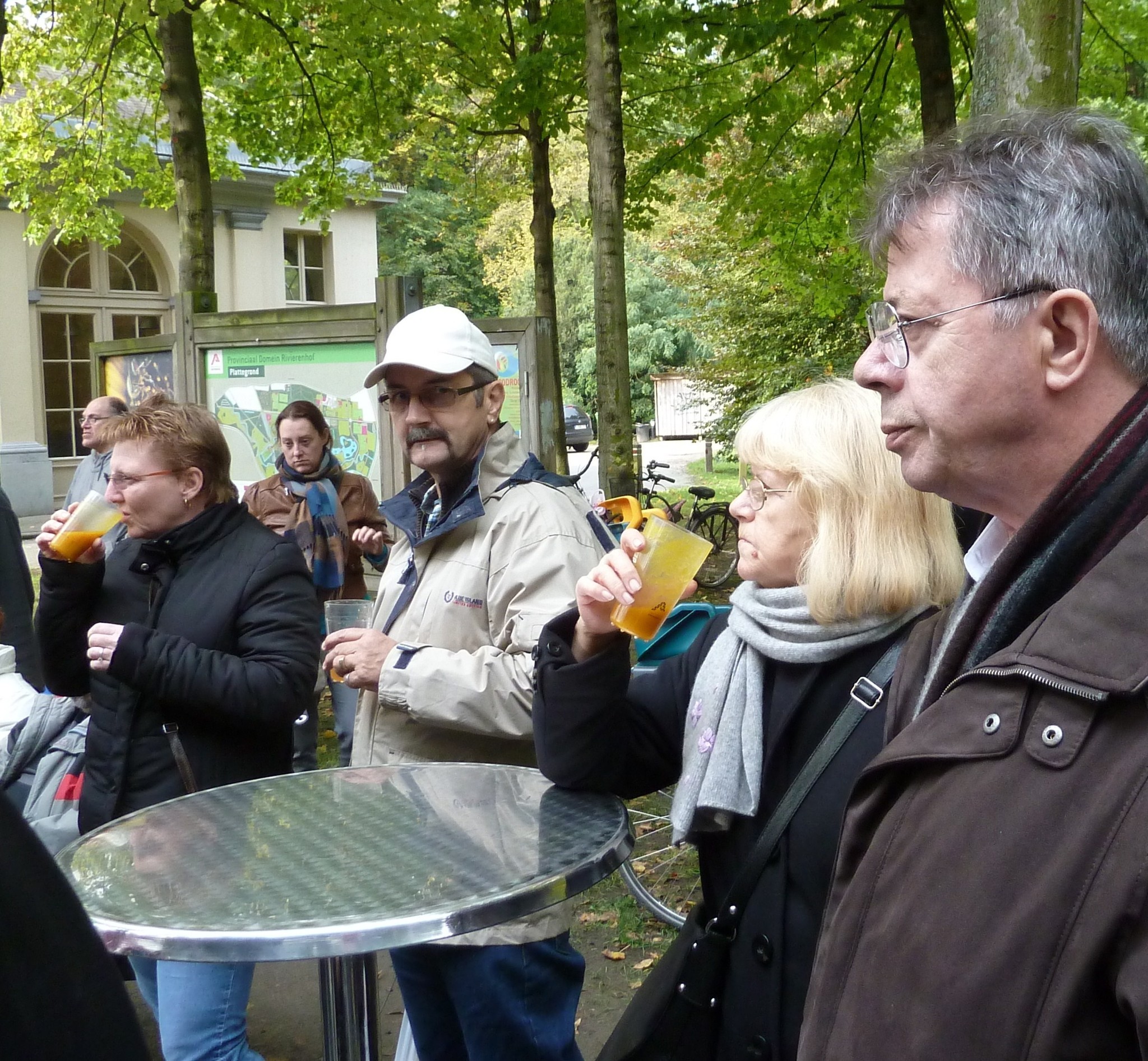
364 304 498 389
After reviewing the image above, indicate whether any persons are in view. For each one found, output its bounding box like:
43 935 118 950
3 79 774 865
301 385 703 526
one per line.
59 396 139 561
0 669 155 1061
29 389 324 1059
319 305 621 1059
0 476 46 695
795 115 1148 1060
239 399 395 776
532 378 976 1061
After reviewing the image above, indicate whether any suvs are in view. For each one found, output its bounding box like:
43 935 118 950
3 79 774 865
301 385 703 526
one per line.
563 403 594 451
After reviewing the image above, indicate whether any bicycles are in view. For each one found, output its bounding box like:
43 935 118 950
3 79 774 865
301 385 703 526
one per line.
609 460 741 589
556 445 599 501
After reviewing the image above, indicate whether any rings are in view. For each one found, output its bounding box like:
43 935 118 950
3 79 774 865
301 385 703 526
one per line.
346 673 355 688
98 647 104 661
339 654 349 671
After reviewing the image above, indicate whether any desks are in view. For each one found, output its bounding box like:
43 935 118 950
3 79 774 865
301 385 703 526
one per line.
53 762 638 1061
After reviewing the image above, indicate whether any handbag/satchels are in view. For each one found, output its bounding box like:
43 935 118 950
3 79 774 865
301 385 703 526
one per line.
596 898 738 1060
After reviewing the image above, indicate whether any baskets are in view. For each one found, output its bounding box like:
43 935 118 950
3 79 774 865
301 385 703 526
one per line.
609 477 635 498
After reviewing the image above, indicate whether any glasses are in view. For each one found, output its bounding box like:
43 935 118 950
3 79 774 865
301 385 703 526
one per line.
378 381 495 412
740 476 797 511
103 470 182 490
79 417 111 425
865 285 1058 369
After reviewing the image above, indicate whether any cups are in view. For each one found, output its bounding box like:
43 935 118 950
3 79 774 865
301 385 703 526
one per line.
48 489 126 564
324 599 373 683
609 514 714 643
